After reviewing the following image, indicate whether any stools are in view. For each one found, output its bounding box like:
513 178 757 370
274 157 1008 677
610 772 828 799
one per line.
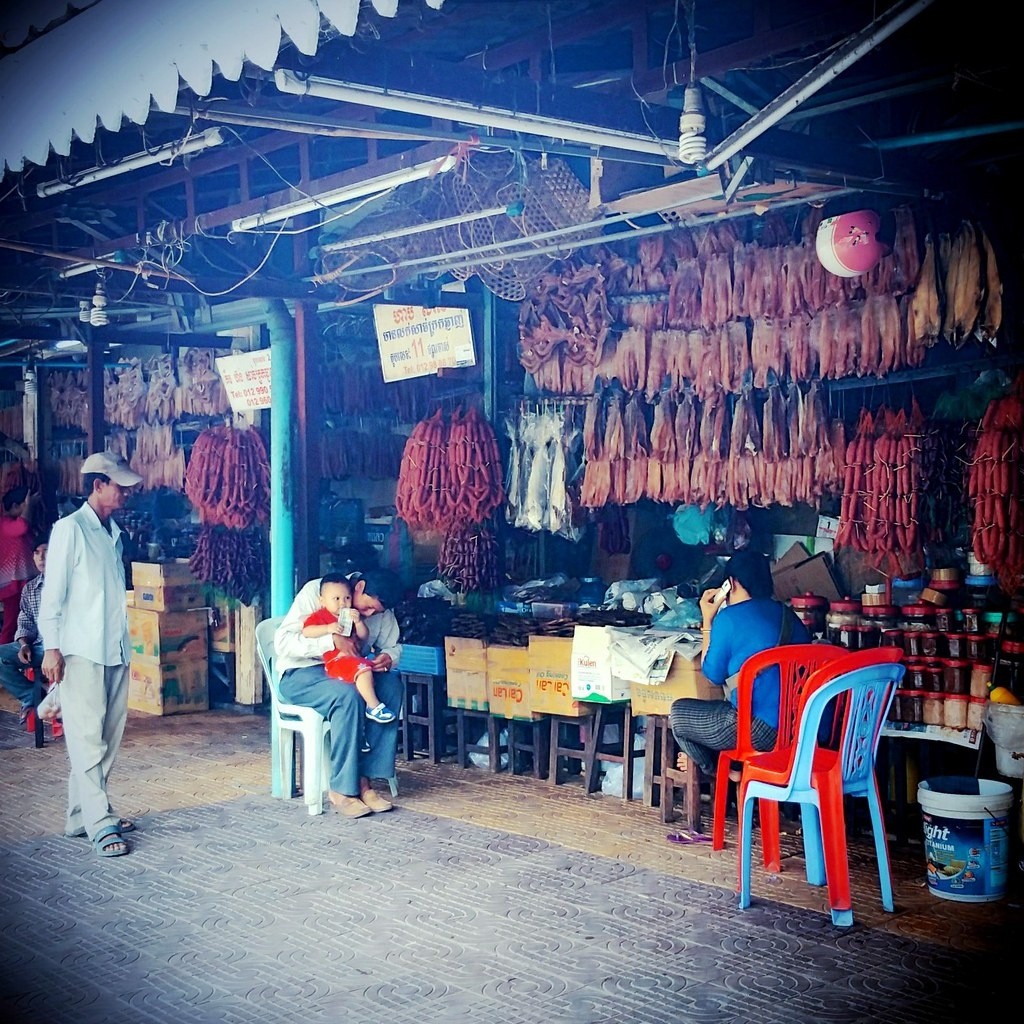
393 669 703 835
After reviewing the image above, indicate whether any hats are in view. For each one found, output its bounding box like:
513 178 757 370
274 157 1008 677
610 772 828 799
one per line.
80 451 144 487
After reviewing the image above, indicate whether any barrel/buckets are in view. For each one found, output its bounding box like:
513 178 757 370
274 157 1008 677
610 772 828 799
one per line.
917 778 1014 902
987 701 1024 778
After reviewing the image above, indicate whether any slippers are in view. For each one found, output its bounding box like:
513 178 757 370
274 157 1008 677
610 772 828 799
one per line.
92 825 129 856
667 827 729 846
66 816 135 837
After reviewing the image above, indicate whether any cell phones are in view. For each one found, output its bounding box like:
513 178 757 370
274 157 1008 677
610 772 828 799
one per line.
713 578 731 604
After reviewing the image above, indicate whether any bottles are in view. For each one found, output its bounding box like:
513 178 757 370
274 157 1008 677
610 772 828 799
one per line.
578 577 607 606
986 681 1021 706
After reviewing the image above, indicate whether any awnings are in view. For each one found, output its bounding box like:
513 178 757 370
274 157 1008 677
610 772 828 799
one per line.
0 0 441 172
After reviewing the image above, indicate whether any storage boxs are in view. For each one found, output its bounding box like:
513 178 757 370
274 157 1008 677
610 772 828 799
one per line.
526 633 596 718
485 644 548 724
395 642 447 678
122 555 236 720
627 647 727 723
443 635 490 714
569 624 649 708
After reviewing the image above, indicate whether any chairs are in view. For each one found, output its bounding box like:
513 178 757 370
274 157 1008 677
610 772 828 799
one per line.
707 642 908 927
255 615 333 816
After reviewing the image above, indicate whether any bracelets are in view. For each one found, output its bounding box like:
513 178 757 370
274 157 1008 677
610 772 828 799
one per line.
701 629 711 633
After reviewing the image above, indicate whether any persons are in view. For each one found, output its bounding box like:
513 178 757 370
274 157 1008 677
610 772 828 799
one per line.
274 569 406 817
37 451 145 857
303 574 396 722
669 548 815 779
0 486 65 728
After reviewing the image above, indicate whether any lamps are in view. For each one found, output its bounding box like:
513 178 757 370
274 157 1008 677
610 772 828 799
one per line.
33 125 226 199
233 155 458 234
273 68 714 169
57 251 123 280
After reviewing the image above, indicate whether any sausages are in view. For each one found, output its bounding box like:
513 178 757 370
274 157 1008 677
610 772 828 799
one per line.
396 406 504 591
184 423 272 591
832 401 920 577
965 396 1024 590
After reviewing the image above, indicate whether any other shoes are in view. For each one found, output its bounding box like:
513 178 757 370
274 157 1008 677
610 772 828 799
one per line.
365 702 396 723
361 788 393 813
330 797 372 819
19 705 33 725
